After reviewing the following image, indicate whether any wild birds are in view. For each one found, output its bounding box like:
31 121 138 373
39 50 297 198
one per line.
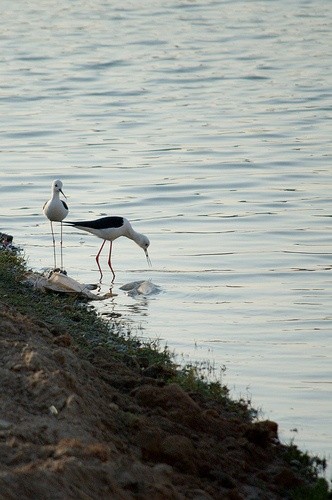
59 216 150 283
42 179 69 234
